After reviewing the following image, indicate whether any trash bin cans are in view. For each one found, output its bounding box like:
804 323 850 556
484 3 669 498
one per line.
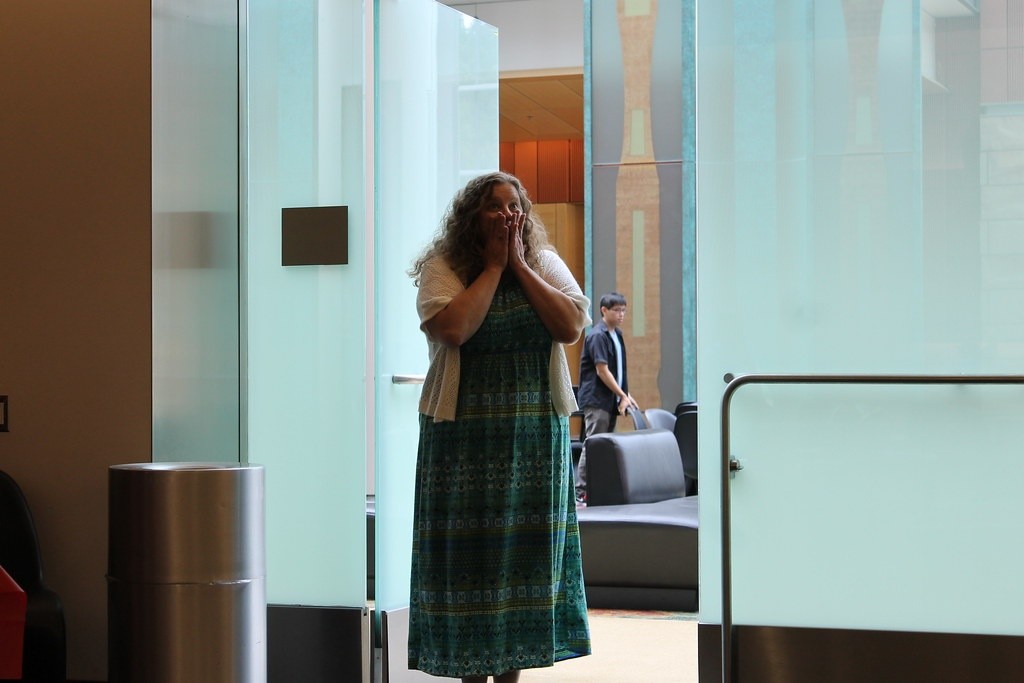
104 461 267 683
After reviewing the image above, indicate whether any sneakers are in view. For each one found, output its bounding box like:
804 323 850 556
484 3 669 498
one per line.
575 495 586 507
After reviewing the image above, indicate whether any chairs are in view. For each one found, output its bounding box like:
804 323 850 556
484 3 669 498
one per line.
628 400 701 494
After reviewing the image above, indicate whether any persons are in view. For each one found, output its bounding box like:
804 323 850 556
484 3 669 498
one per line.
405 172 593 683
575 292 640 508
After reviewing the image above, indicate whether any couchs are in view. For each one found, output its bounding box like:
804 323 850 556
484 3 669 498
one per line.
570 427 698 613
